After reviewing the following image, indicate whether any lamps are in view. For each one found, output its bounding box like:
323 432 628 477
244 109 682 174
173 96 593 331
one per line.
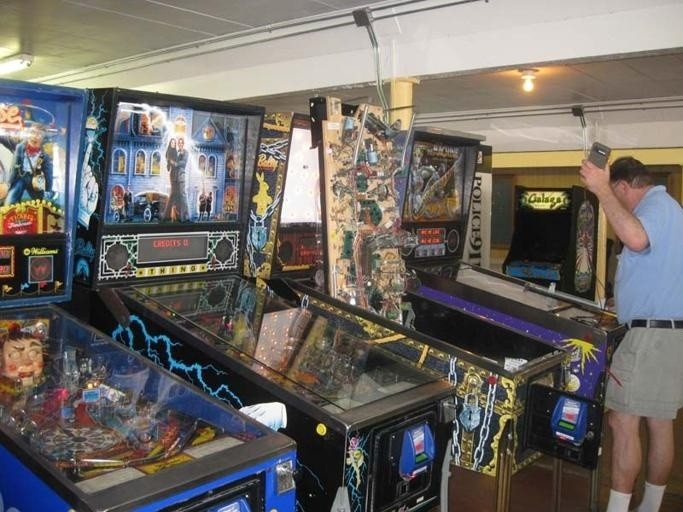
518 67 539 91
0 54 33 76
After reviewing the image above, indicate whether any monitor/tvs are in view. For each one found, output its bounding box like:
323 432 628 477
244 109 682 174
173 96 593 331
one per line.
519 213 571 263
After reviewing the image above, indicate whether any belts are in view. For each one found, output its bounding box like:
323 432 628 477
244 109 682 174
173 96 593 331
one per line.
624 319 683 331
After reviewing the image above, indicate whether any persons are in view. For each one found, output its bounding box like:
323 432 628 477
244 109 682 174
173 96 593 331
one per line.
160 140 182 224
205 191 212 220
168 140 191 221
196 192 205 221
578 156 683 512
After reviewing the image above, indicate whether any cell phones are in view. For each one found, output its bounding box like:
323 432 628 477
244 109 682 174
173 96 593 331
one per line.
589 142 611 169
